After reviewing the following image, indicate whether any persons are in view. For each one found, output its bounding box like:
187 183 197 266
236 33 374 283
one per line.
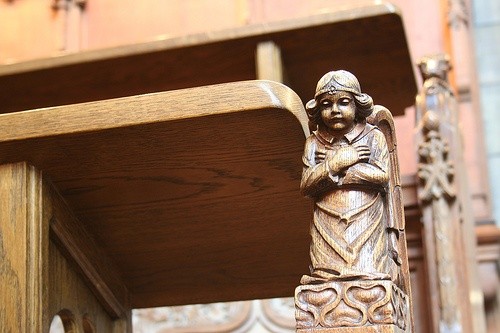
301 70 398 284
